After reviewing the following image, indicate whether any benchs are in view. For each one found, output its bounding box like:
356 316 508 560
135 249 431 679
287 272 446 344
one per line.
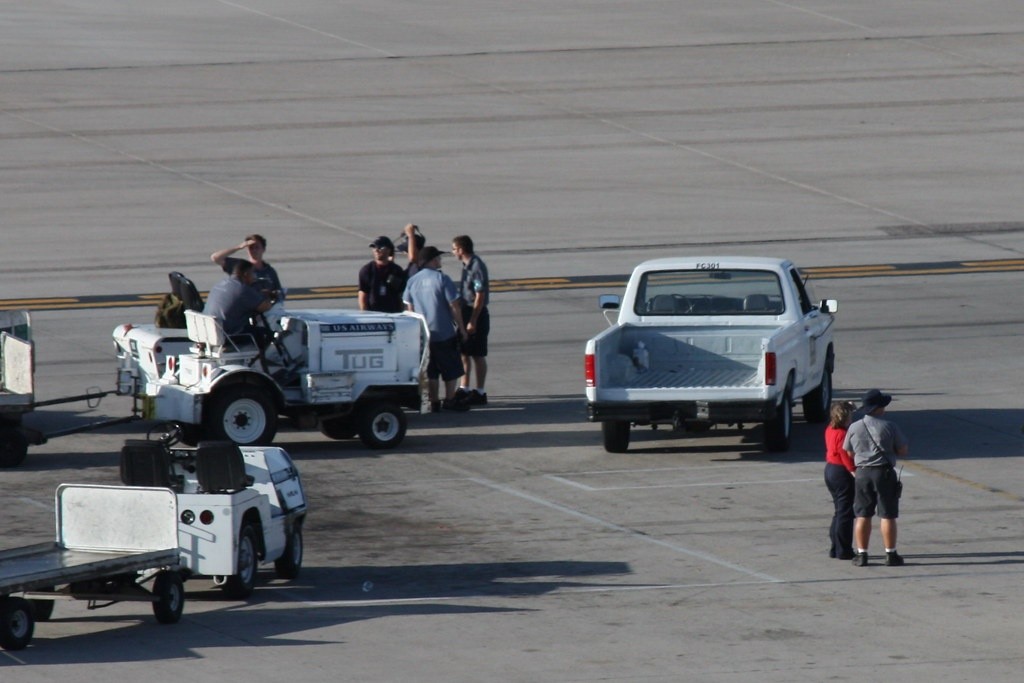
184 310 262 358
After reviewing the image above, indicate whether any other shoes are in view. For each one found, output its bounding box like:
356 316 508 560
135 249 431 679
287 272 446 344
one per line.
442 399 463 409
429 401 444 413
852 550 868 566
454 387 488 405
886 550 903 566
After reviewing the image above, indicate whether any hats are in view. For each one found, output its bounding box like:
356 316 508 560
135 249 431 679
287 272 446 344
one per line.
369 236 393 250
418 246 451 268
859 389 892 414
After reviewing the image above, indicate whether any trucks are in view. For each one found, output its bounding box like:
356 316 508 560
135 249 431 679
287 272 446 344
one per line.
0 429 308 651
0 271 431 470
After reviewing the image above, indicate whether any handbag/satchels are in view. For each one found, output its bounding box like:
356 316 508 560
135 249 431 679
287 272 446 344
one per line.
155 292 189 330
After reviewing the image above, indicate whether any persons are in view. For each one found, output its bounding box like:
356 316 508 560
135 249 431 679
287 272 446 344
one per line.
200 224 490 411
842 389 904 566
211 235 284 311
824 400 856 560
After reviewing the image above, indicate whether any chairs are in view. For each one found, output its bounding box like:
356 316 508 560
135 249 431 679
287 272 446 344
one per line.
193 443 256 489
119 440 175 487
744 294 777 313
647 294 685 315
164 272 205 312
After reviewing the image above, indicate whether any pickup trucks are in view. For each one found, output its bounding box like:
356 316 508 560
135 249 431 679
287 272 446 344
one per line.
582 254 839 452
